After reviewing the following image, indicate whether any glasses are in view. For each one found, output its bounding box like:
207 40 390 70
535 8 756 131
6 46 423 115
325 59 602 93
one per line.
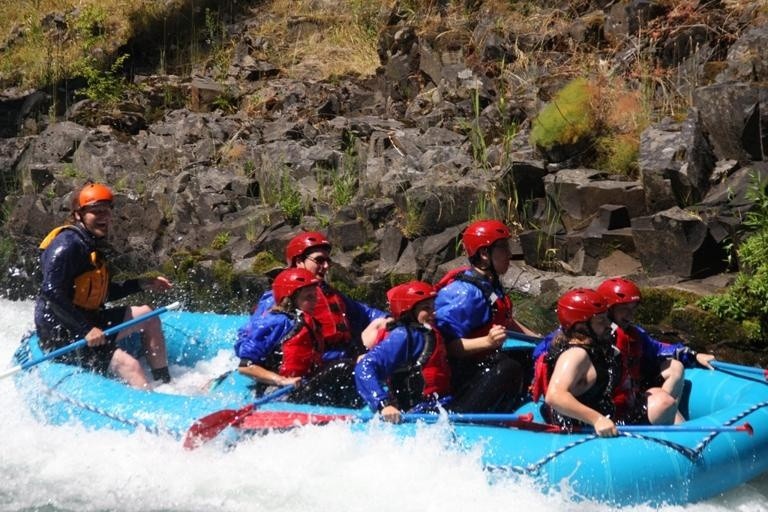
309 255 333 265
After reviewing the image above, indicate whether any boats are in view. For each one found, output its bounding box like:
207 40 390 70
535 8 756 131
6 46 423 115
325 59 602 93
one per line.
14 309 768 507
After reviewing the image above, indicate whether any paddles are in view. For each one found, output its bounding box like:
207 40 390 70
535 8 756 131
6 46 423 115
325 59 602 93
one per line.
502 421 753 438
230 412 533 430
181 377 312 450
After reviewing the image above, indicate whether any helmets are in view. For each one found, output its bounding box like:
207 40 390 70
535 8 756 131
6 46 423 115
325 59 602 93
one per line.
386 279 439 320
271 267 321 306
69 180 113 217
556 287 608 332
285 231 332 271
463 219 512 259
598 276 643 307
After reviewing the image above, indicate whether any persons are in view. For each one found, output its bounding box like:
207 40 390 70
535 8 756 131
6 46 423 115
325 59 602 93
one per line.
355 279 524 424
432 219 544 357
250 232 393 363
532 278 717 423
237 270 356 409
532 288 678 437
34 183 175 391
